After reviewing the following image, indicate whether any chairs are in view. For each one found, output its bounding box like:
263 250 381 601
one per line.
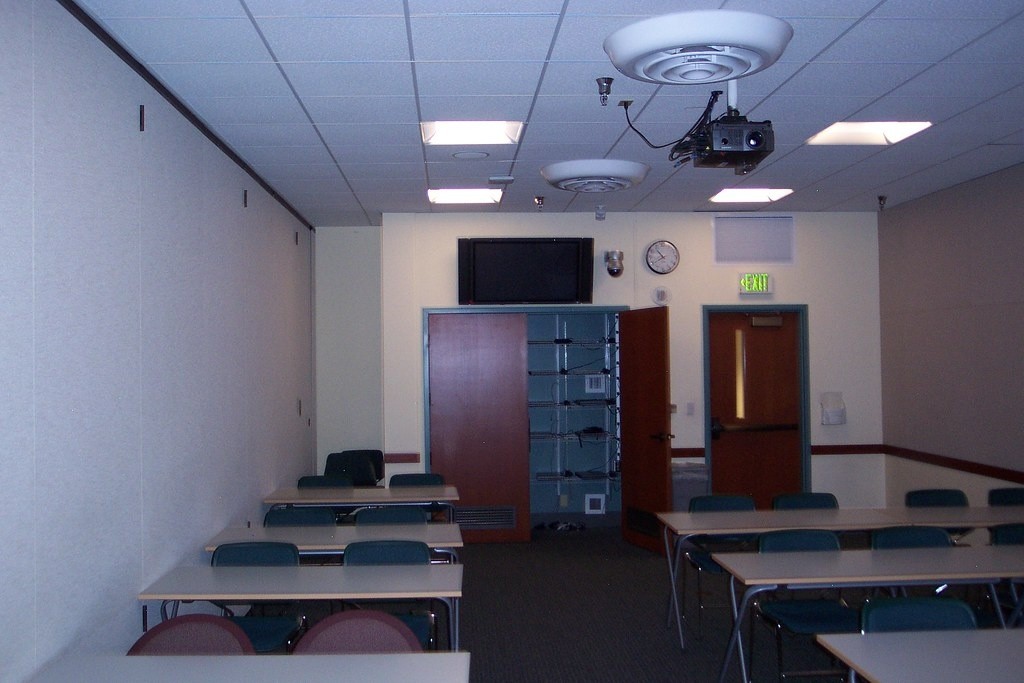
354 506 434 525
771 490 840 511
869 525 1010 629
993 521 1024 627
297 474 356 487
681 494 763 640
124 612 256 656
333 538 437 648
850 594 978 683
986 486 1024 544
293 608 423 656
343 446 386 487
263 506 338 528
388 472 446 490
323 451 377 486
747 529 859 683
207 540 305 652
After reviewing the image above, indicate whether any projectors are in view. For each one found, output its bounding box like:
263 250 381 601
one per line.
694 120 774 176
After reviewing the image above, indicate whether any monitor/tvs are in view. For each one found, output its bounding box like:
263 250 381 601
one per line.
458 237 594 305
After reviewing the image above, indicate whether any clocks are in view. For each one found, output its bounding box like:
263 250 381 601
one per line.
644 238 683 275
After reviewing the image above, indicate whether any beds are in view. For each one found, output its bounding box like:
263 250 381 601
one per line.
904 486 980 550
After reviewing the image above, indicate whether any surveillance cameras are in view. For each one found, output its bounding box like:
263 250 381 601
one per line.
605 250 624 277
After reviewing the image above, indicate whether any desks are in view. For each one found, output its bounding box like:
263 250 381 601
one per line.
22 651 474 683
707 541 1024 683
813 624 1024 683
203 519 464 565
882 503 1024 541
137 562 466 649
652 509 914 658
259 481 460 522
